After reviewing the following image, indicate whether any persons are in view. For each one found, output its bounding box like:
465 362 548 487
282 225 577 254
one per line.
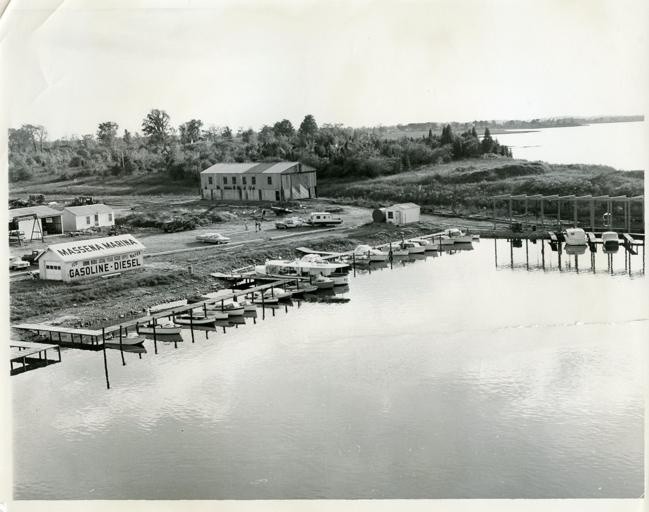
243 218 248 230
254 213 263 232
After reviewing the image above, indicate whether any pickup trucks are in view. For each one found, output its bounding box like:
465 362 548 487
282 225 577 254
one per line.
275 218 302 230
9 250 44 271
196 232 231 246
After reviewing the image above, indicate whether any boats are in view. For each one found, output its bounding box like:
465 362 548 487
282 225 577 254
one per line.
105 283 320 346
303 212 343 225
351 227 481 265
601 231 619 248
563 228 588 246
254 259 351 286
